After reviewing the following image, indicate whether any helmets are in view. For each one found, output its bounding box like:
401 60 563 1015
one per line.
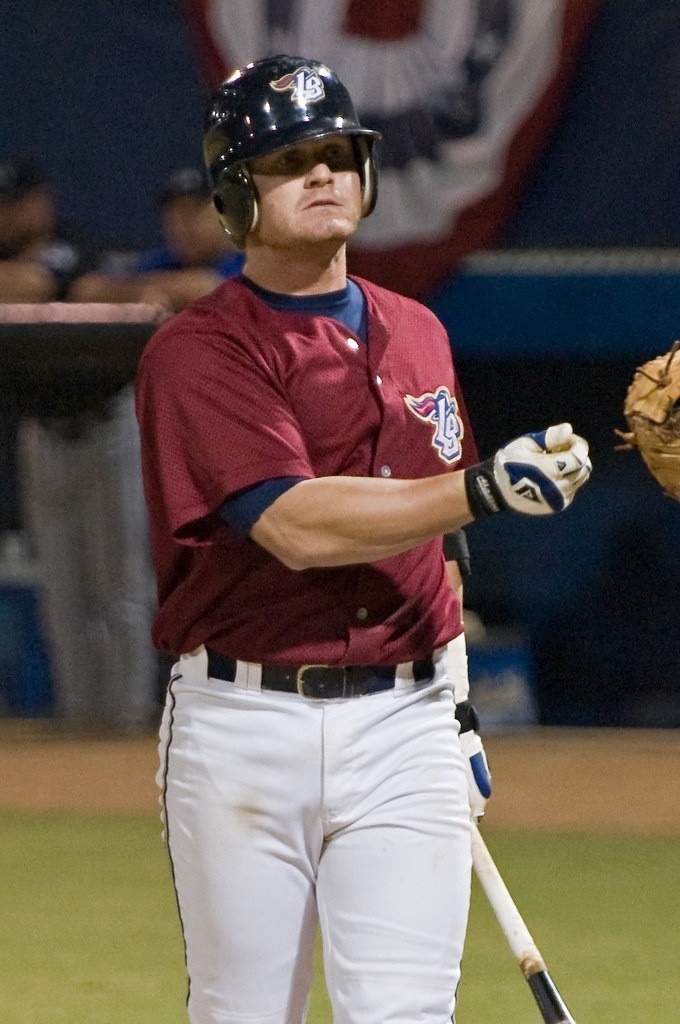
199 55 383 246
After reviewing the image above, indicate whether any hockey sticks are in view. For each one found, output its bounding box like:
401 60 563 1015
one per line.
473 826 576 1024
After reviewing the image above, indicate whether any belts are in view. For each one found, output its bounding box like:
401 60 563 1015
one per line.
206 650 433 703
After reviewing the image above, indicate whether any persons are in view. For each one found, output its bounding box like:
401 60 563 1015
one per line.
132 54 590 1024
0 164 248 734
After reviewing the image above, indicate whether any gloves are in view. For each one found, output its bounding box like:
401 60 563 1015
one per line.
464 422 593 524
453 700 493 825
